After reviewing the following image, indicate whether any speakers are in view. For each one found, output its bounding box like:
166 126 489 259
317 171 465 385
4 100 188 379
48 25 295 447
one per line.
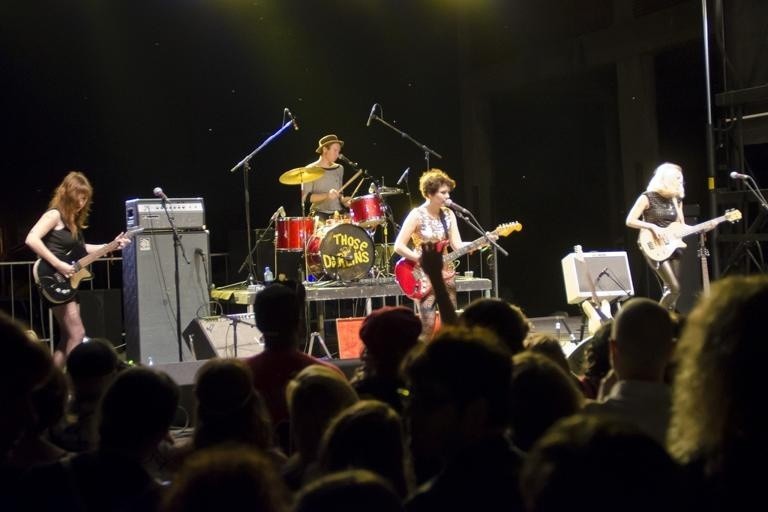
183 312 267 361
567 335 595 379
561 250 635 305
325 358 368 382
147 358 212 430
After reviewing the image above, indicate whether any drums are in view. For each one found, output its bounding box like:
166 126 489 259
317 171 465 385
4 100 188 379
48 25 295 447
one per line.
275 217 315 253
349 193 385 227
305 222 374 283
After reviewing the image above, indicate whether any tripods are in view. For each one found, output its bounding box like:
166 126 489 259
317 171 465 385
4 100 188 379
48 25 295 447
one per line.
374 194 396 280
317 248 353 286
215 120 291 290
297 176 319 289
368 229 386 281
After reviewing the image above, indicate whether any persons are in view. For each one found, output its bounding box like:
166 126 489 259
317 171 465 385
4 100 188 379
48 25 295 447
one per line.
2 277 767 512
393 169 500 339
24 172 132 367
302 134 352 228
624 163 719 311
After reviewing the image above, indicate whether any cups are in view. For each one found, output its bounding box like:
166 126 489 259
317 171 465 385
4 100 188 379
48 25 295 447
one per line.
574 245 583 253
464 271 474 278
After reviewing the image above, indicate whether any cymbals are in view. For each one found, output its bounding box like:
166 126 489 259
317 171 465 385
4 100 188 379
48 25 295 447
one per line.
372 187 405 195
279 166 325 185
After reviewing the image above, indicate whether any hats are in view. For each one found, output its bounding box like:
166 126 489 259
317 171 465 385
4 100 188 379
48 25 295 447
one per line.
360 306 422 351
315 134 344 154
66 338 131 378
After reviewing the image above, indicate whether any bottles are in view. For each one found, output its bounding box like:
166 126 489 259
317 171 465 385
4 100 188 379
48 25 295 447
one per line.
263 266 273 281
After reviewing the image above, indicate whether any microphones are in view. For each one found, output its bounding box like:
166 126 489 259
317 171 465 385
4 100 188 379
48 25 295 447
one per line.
339 154 353 165
153 187 170 203
367 104 377 127
730 171 748 179
346 255 353 261
595 266 608 282
396 167 410 185
444 199 471 214
286 108 299 130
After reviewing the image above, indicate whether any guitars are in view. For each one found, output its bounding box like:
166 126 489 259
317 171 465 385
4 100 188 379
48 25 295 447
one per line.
395 220 523 299
33 225 145 303
638 208 742 262
